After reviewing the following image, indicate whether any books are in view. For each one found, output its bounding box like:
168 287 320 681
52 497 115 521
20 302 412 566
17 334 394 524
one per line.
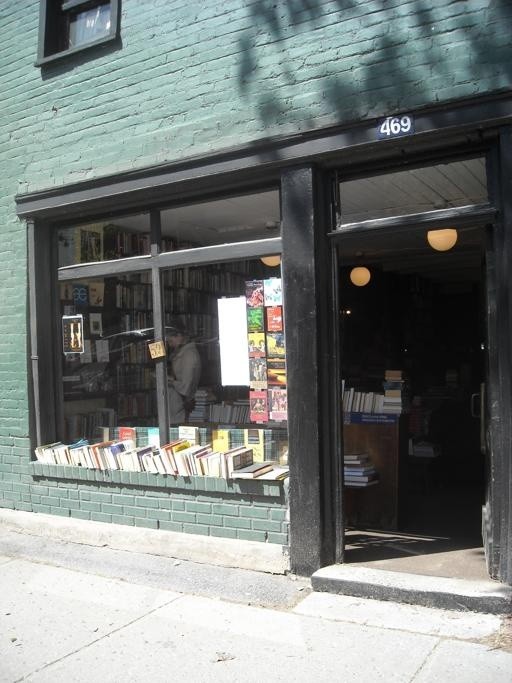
340 369 460 488
57 225 264 445
244 277 288 422
34 425 290 482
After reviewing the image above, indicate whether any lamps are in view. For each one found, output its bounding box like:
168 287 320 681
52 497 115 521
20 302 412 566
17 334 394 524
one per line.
426 198 458 252
